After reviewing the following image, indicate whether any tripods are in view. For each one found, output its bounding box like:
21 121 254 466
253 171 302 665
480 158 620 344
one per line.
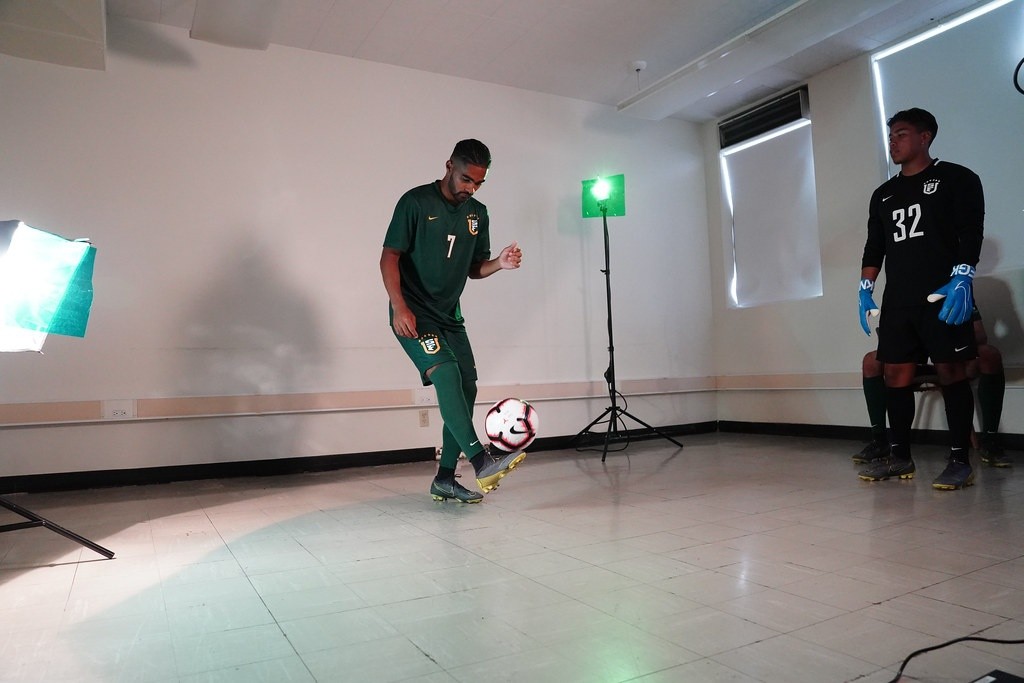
573 202 684 462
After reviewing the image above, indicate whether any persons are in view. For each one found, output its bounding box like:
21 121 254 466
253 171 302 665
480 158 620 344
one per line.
850 297 1015 468
859 108 985 490
378 139 525 505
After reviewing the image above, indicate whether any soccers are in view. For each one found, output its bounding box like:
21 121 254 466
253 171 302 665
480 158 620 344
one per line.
485 397 538 453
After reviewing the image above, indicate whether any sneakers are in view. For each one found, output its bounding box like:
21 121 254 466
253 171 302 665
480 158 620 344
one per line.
933 456 976 490
475 451 528 495
857 456 916 480
430 476 482 504
852 444 892 464
978 443 1013 467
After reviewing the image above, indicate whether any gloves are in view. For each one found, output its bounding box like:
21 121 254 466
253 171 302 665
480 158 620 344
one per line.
927 261 980 325
857 277 879 336
